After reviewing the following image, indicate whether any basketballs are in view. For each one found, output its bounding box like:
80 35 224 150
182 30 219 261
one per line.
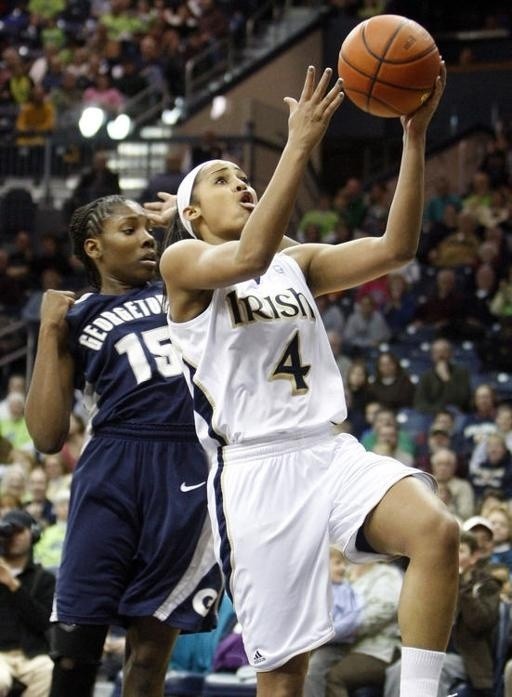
337 15 441 118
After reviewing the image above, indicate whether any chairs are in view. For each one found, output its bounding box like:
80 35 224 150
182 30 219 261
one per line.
360 260 511 441
446 592 510 697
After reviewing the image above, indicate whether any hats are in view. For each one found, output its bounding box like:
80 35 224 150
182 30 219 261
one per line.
462 517 494 540
0 509 40 545
5 466 50 491
427 419 454 437
